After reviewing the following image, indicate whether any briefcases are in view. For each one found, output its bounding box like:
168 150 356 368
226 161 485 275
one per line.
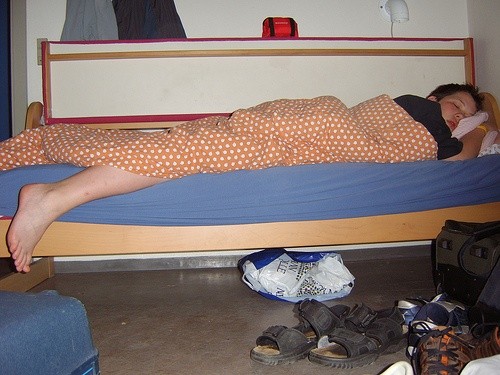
435 219 500 320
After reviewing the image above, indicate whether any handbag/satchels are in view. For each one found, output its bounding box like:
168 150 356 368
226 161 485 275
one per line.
262 16 298 38
237 246 355 302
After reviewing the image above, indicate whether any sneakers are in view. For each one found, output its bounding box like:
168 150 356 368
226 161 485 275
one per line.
396 294 500 375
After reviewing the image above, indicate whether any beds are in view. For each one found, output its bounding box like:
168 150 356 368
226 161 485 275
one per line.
0 38 499 292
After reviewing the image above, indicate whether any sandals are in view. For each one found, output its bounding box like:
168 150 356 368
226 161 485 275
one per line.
309 304 411 369
250 298 350 367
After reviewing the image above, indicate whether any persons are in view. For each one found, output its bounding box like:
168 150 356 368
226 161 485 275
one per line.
6 83 493 274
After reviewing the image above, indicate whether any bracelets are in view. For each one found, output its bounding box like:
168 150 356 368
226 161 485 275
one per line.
478 123 488 133
